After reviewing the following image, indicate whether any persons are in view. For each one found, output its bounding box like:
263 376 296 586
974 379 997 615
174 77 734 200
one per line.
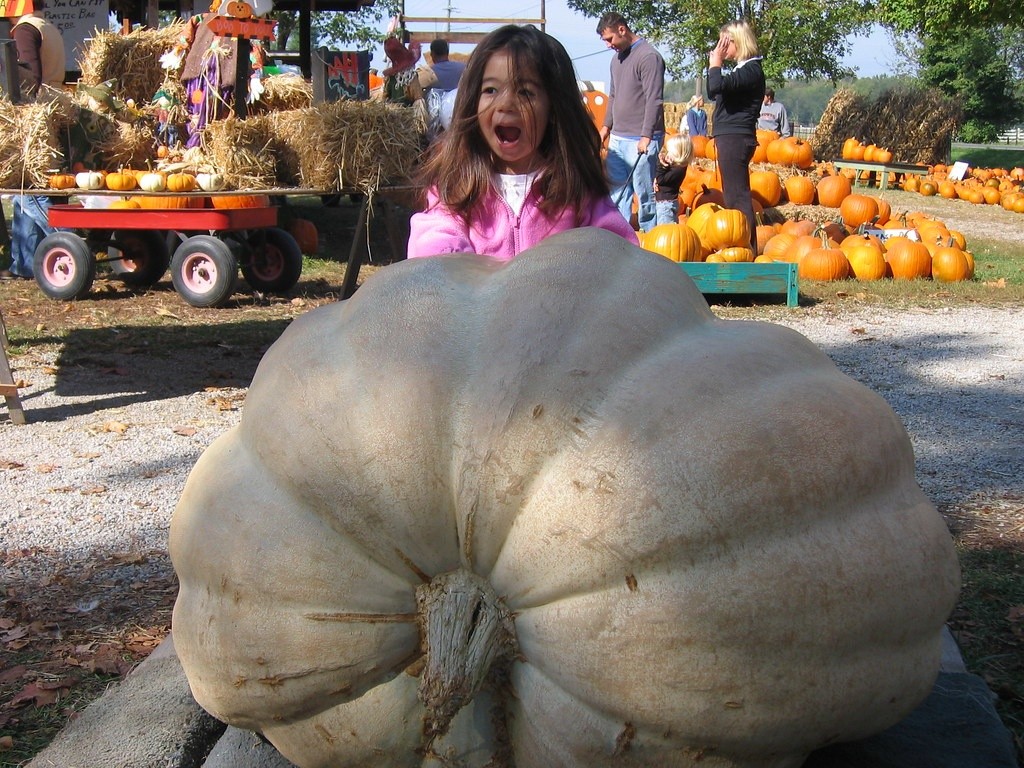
679 94 709 139
703 20 765 261
406 22 642 262
0 0 74 280
756 86 792 138
593 11 666 235
653 135 692 224
424 38 470 92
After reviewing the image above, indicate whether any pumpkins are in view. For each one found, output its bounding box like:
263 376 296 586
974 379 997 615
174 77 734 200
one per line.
601 128 1024 282
280 212 318 255
48 140 271 210
369 68 383 90
165 227 959 768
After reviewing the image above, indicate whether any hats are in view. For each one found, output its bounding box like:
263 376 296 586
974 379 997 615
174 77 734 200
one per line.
382 36 422 76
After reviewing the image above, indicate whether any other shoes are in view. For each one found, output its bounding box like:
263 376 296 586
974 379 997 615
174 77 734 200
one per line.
0 268 19 281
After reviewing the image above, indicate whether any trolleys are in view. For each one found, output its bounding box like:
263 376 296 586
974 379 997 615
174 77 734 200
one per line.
31 202 306 308
